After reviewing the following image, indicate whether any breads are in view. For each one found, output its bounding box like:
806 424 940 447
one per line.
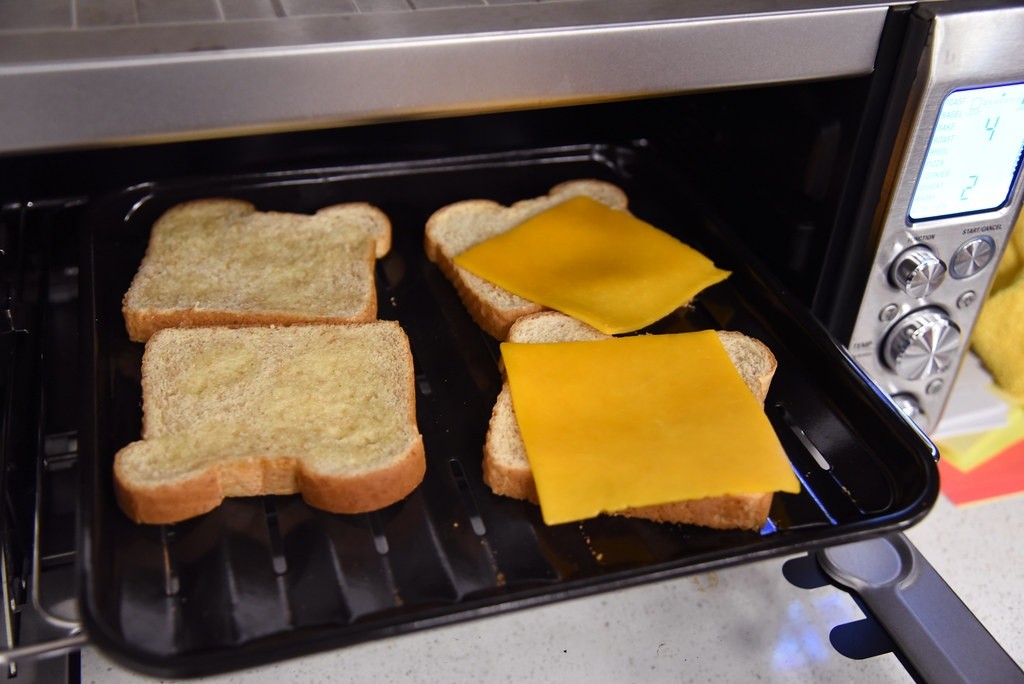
122 198 392 342
114 319 427 524
426 179 636 339
480 309 778 532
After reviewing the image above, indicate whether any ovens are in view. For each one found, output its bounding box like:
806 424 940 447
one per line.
0 1 1024 684
